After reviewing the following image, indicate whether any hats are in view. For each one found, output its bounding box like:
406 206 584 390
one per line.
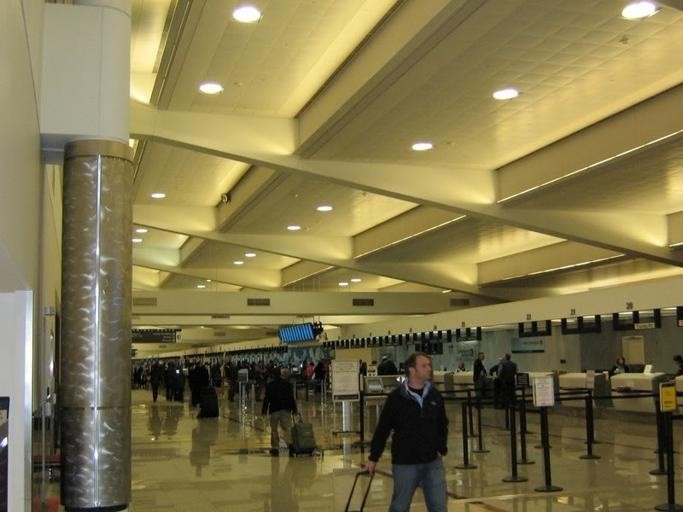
383 356 387 359
271 368 281 376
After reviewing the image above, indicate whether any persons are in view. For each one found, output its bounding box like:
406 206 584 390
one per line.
369 351 449 512
377 356 397 375
132 355 330 418
473 352 487 408
612 356 629 375
262 367 299 456
673 355 683 377
490 354 517 407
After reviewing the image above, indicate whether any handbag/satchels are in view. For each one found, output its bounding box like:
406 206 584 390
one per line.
200 388 217 398
174 384 184 391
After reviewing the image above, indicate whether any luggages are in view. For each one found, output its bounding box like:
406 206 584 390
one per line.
291 412 316 456
174 392 183 402
200 398 219 418
